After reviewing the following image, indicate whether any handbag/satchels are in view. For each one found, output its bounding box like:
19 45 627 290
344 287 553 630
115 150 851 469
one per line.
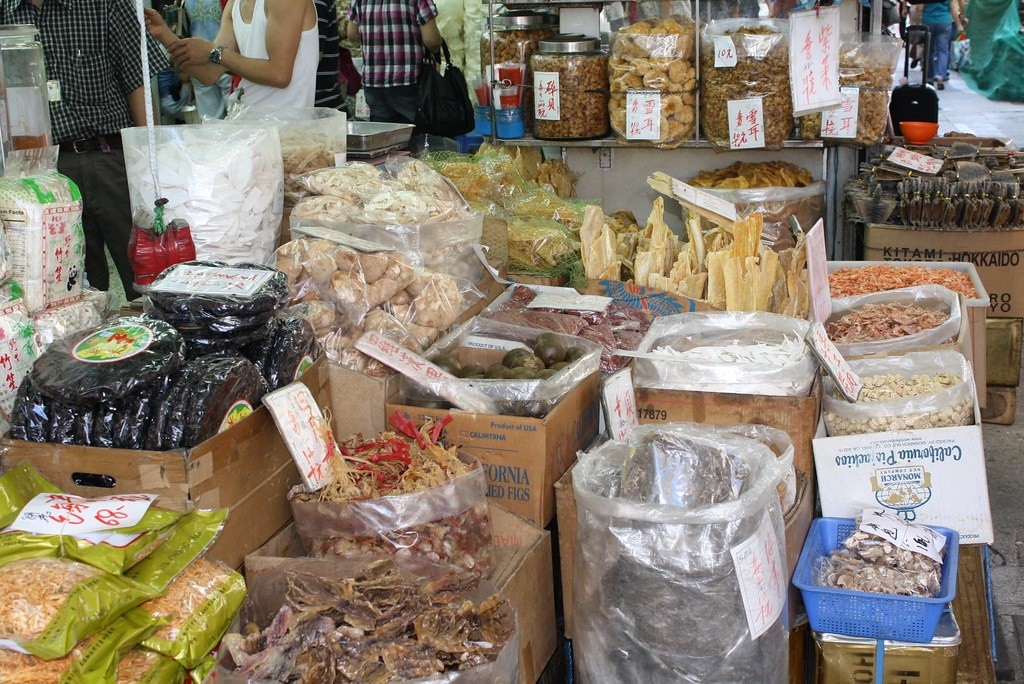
418 38 475 136
163 0 189 38
950 34 972 69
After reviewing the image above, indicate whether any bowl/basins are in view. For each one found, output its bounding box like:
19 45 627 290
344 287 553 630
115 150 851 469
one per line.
900 122 939 145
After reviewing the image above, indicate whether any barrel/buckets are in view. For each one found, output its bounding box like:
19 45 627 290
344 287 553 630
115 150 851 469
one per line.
986 317 1024 387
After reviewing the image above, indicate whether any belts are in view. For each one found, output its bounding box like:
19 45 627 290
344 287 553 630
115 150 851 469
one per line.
56 134 122 154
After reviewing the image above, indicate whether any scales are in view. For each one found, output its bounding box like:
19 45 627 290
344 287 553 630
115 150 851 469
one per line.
346 121 415 170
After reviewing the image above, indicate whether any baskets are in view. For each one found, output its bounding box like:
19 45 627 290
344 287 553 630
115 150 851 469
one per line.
792 517 959 644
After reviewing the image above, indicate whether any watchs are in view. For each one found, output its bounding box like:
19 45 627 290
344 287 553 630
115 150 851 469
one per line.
209 45 226 64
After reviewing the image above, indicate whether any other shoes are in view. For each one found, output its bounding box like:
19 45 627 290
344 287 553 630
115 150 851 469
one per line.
911 57 921 68
937 76 944 90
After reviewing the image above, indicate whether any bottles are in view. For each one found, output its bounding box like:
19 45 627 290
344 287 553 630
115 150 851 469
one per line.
529 33 611 141
497 106 525 138
474 103 493 135
0 24 53 160
479 10 560 133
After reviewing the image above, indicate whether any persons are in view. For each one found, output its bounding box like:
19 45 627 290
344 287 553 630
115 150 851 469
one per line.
0 0 171 299
314 0 350 120
890 0 966 90
347 0 442 150
182 0 241 121
144 0 319 112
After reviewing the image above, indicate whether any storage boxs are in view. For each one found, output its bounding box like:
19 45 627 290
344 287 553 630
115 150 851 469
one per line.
0 136 1024 684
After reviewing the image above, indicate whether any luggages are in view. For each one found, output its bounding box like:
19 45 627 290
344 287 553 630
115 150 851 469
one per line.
890 25 939 135
